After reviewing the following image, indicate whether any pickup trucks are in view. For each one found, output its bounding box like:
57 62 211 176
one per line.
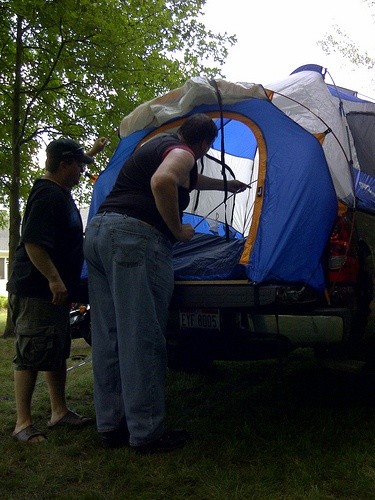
65 63 374 365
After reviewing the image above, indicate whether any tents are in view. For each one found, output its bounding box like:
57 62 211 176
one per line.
80 63 375 304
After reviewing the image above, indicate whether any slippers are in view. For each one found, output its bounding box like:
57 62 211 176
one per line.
47 410 93 430
11 425 48 444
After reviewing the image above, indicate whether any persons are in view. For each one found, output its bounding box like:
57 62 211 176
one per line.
6 136 109 445
82 112 252 453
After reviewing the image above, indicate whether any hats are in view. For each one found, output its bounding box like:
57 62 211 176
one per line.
46 137 94 164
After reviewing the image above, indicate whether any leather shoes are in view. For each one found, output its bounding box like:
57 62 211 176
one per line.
101 430 131 448
129 432 192 452
